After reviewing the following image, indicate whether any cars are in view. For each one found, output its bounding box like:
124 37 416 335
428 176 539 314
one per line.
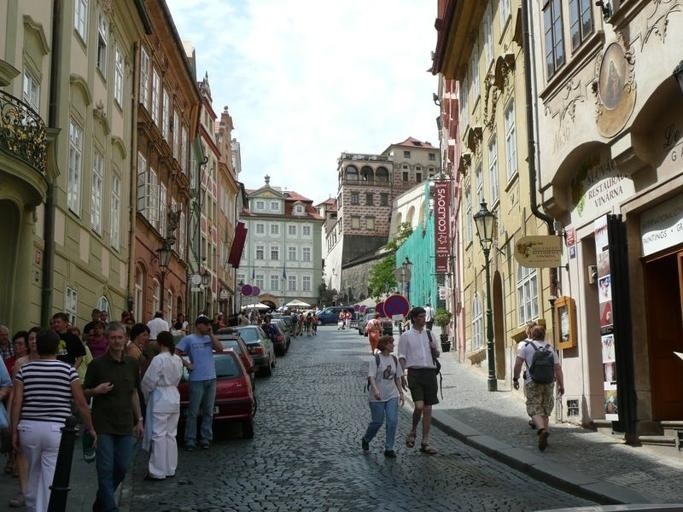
256 314 299 356
358 313 393 336
229 324 276 379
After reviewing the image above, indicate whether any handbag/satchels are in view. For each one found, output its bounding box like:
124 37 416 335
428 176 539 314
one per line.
426 330 443 400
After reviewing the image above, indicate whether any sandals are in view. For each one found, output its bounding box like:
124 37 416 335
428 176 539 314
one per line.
12 464 19 478
406 430 416 448
10 491 26 506
5 459 14 473
419 441 438 454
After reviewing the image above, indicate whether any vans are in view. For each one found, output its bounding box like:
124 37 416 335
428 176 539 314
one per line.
315 306 354 325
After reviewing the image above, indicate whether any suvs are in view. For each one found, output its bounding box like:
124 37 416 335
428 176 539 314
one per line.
177 348 257 440
364 306 375 315
211 330 254 397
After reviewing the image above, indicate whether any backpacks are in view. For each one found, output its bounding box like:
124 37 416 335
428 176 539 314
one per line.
367 354 397 391
526 341 556 384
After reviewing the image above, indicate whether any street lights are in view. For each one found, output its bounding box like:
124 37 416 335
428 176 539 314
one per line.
473 197 499 393
401 256 413 334
384 279 390 298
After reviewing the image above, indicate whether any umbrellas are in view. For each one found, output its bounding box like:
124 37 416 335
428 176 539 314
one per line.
286 299 311 307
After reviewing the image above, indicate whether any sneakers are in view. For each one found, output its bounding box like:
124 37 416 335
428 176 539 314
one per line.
144 474 165 480
537 429 550 451
200 439 209 449
74 426 80 438
362 436 369 450
528 419 535 429
83 449 97 462
384 449 398 459
184 440 196 451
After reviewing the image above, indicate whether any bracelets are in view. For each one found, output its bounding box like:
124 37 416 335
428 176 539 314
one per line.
513 377 517 381
400 392 403 393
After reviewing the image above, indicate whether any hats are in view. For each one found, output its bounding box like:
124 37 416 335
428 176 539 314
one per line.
196 314 215 325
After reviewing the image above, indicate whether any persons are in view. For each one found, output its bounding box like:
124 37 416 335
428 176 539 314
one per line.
292 308 410 356
362 333 404 458
0 303 279 512
424 303 436 330
513 325 564 451
517 322 537 429
398 306 440 454
